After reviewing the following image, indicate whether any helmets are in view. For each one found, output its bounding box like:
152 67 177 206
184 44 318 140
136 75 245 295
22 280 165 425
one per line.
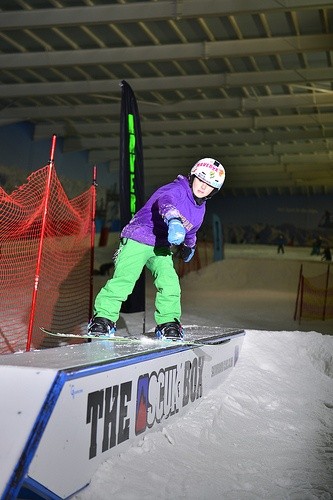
190 157 226 191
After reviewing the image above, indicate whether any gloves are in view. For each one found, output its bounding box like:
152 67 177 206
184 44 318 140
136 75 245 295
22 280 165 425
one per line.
165 216 186 245
182 247 195 262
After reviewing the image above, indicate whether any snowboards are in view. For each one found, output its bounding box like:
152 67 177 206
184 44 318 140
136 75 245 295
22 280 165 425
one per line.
39 326 231 345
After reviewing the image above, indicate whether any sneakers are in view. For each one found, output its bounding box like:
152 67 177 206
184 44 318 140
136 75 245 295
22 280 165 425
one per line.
155 321 184 340
87 315 117 335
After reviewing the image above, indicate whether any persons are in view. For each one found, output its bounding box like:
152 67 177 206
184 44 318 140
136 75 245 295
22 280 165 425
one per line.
88 157 226 338
310 236 322 256
277 235 284 254
321 247 332 261
